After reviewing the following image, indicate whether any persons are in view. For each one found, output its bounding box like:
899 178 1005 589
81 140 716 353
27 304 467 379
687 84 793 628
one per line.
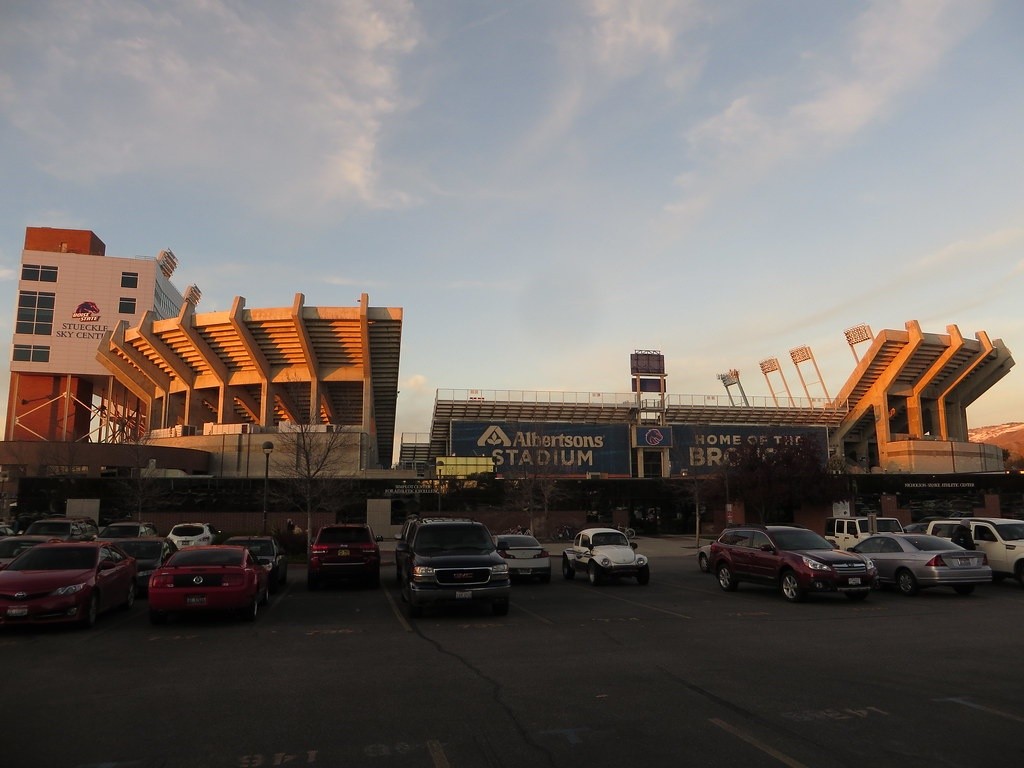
287 519 295 530
951 520 975 549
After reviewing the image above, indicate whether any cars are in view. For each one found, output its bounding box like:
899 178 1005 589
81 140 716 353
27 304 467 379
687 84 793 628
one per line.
927 516 1024 587
903 516 942 535
308 524 384 589
96 522 160 541
561 527 650 588
0 541 137 626
695 542 714 572
22 515 100 543
826 516 904 552
148 545 270 622
0 536 64 571
494 535 552 583
222 535 288 596
167 523 221 549
0 524 17 536
846 533 993 596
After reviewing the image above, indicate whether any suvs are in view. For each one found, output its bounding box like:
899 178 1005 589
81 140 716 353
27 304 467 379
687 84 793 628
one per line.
395 513 511 616
705 522 880 604
